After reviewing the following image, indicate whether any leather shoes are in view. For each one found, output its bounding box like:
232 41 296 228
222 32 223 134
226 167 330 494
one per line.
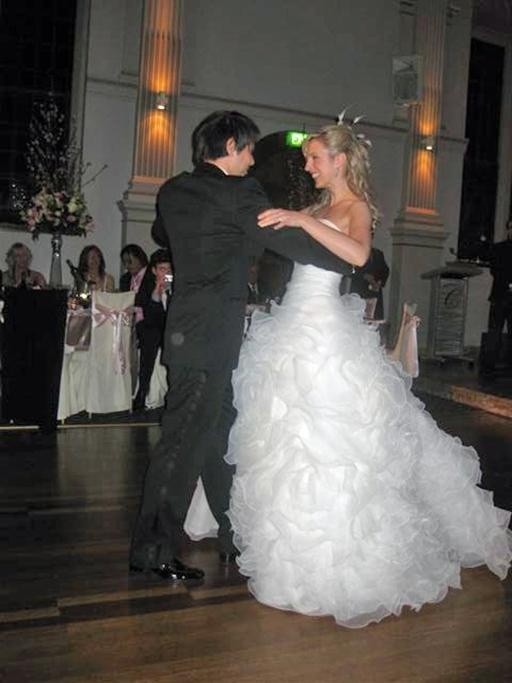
129 557 205 581
218 551 241 563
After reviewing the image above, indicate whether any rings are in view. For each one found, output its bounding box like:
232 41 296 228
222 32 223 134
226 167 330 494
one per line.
276 216 281 221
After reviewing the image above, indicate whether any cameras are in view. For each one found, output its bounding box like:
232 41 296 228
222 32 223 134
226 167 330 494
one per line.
165 273 174 283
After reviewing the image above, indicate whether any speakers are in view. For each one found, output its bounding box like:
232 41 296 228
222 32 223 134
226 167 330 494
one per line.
392 54 424 108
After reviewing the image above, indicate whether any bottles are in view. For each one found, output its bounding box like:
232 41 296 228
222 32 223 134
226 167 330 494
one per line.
65 260 89 292
17 271 27 289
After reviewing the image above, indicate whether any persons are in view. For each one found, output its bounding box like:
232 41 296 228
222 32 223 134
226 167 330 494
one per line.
68 244 114 310
127 109 354 579
349 226 390 321
134 248 175 409
1 243 47 289
479 219 512 378
183 118 512 631
120 243 154 348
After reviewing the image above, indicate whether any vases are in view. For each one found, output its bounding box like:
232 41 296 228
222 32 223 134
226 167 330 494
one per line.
47 232 65 291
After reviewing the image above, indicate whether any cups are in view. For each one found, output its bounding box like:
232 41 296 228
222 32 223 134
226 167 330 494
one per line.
67 298 77 311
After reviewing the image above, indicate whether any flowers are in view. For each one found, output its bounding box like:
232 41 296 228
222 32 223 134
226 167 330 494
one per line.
9 88 112 245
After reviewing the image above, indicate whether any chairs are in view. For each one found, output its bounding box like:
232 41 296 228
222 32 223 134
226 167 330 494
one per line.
63 289 138 418
